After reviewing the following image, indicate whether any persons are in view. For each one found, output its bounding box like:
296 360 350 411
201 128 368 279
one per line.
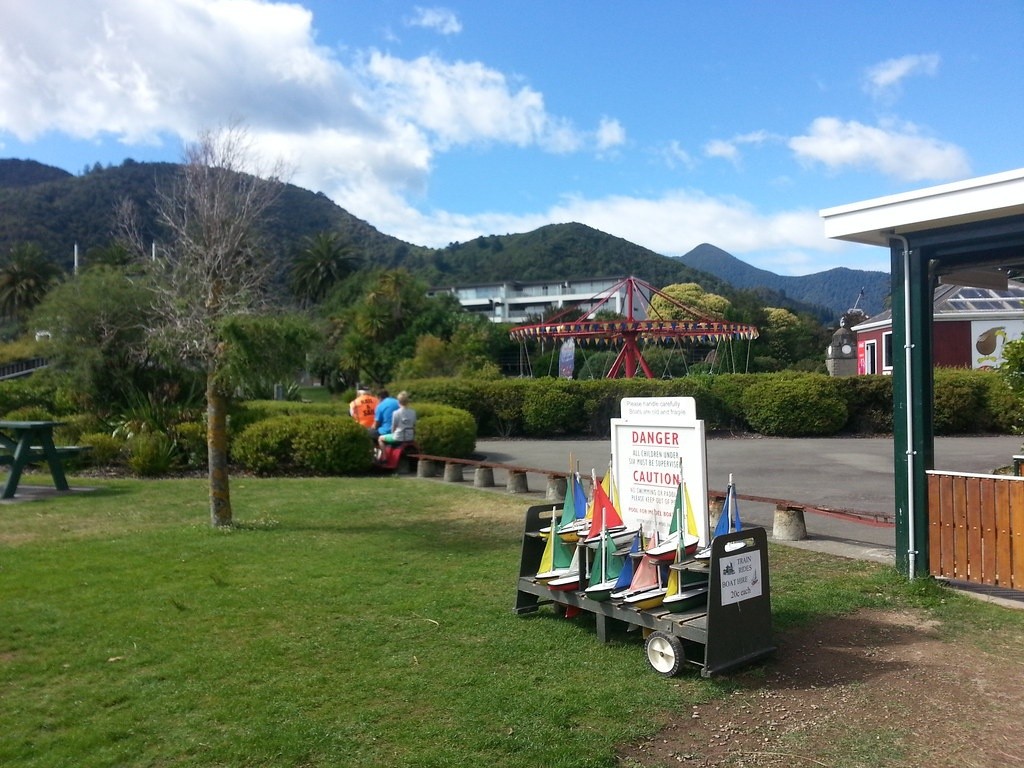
704 342 720 371
347 384 417 462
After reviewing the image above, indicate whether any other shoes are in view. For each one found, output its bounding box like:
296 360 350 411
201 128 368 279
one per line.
378 459 387 464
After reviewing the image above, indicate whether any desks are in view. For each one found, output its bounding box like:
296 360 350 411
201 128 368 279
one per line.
0 422 70 501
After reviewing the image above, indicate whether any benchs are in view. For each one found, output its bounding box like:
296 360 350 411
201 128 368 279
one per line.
0 445 94 466
400 449 896 567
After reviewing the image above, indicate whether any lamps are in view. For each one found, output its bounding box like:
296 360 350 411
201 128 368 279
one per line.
1006 269 1023 281
841 336 852 355
